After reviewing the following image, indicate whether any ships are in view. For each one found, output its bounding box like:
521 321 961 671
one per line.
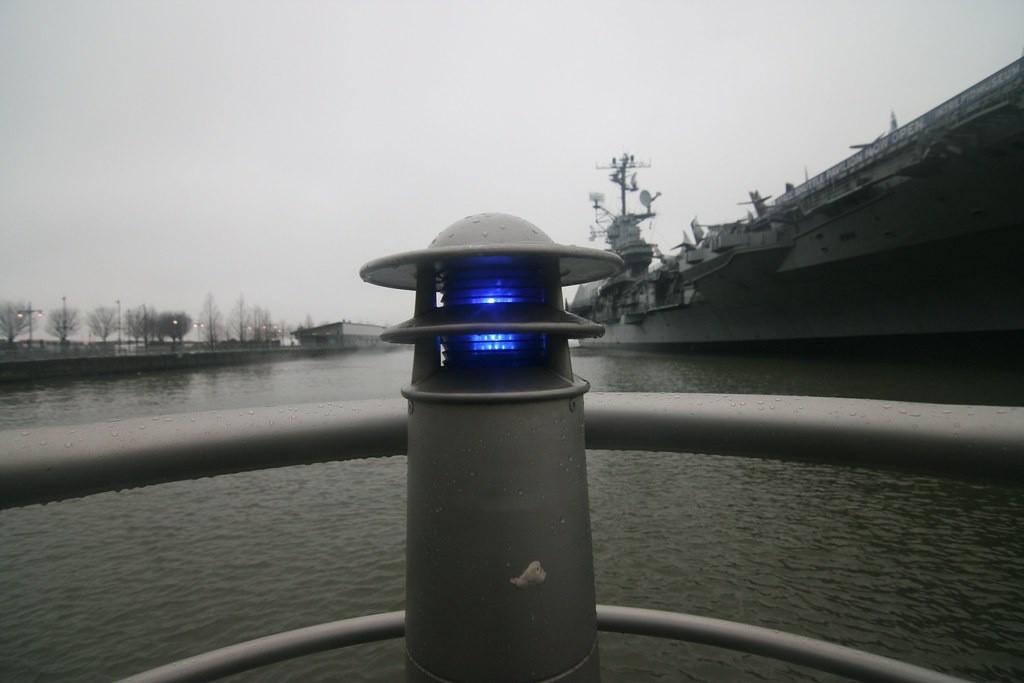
569 51 1023 349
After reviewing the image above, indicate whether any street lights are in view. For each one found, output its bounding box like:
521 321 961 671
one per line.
172 319 178 351
194 322 204 351
16 301 46 348
